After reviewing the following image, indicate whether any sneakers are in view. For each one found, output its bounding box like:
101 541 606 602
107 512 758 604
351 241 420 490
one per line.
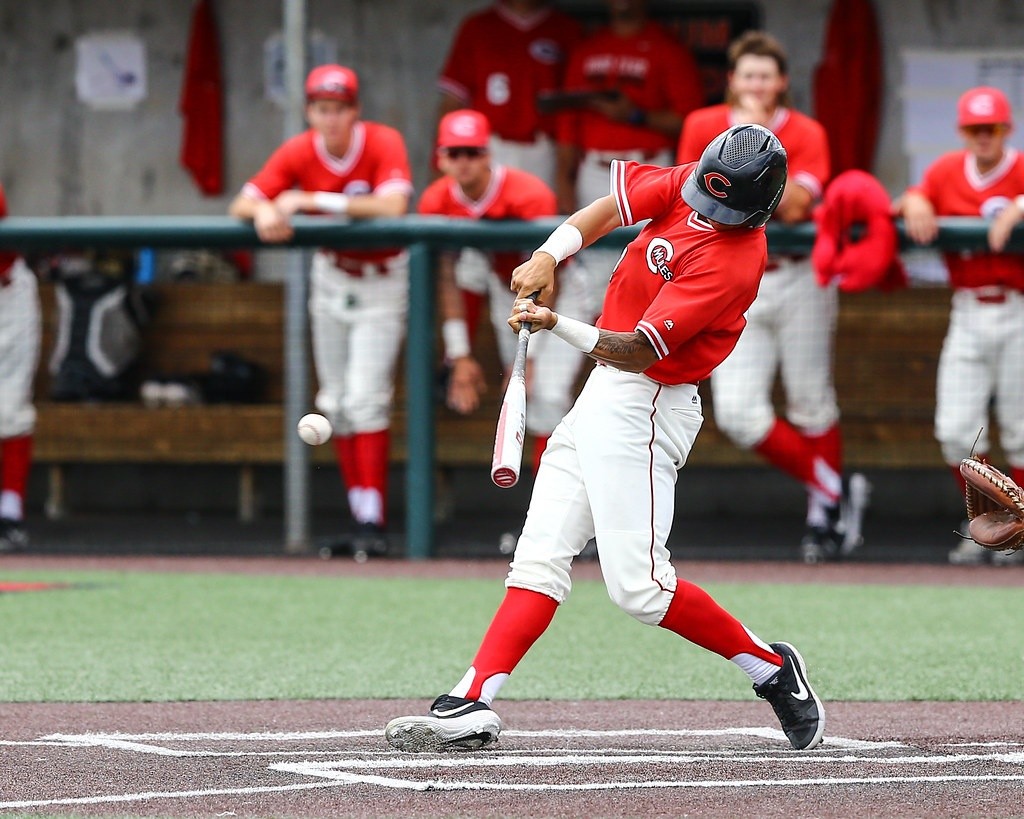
385 694 502 753
753 641 826 750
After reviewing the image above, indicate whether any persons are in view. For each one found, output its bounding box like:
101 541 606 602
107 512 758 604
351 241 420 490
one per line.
381 123 827 754
892 86 1023 565
674 35 873 565
228 62 410 558
436 0 703 466
415 111 600 558
1 183 45 552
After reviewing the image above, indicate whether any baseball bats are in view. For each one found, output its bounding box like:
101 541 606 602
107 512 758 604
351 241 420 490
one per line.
490 292 537 489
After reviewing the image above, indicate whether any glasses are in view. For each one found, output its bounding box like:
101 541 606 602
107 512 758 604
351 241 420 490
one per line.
447 145 484 160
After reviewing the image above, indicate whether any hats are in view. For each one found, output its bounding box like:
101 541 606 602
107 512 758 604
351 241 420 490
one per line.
306 64 359 108
958 86 1012 134
440 108 492 148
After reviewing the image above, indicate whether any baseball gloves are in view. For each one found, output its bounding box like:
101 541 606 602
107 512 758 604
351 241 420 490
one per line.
960 458 1024 551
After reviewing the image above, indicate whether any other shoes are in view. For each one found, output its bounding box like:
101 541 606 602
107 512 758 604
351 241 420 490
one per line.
828 471 867 557
802 496 841 563
0 515 30 550
948 540 1024 564
326 521 391 556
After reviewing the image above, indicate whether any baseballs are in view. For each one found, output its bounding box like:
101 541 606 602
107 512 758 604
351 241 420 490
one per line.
297 414 332 445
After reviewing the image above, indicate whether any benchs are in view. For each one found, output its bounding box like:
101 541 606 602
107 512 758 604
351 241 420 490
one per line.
30 284 404 529
433 288 1005 526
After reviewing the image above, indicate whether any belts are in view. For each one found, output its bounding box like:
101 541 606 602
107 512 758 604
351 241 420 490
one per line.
765 254 807 271
600 150 660 167
976 284 1008 304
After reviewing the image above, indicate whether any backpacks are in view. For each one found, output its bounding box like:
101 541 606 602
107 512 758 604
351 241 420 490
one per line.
40 259 156 404
